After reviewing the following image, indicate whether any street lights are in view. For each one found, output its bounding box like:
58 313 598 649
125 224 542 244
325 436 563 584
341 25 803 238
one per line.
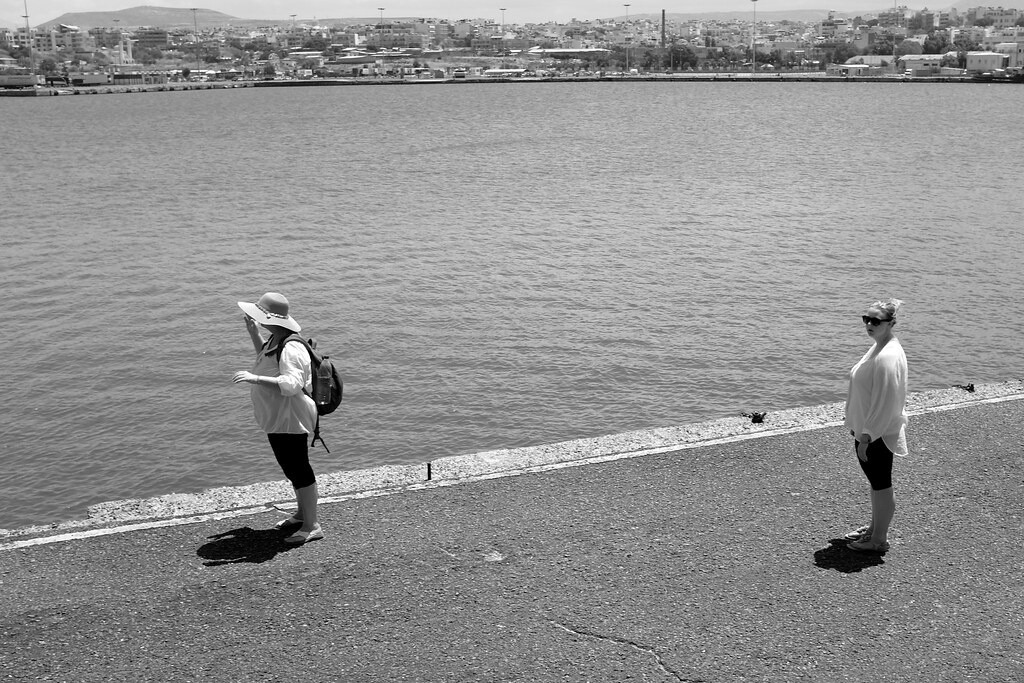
113 18 121 64
623 2 631 73
21 15 30 74
290 13 296 49
378 7 385 52
751 0 758 76
500 8 507 71
189 8 200 73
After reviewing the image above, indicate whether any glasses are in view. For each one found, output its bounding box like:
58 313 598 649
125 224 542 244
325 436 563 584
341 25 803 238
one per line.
862 315 889 326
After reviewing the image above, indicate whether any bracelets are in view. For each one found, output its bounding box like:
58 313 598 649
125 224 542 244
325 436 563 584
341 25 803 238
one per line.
257 374 261 384
860 434 871 444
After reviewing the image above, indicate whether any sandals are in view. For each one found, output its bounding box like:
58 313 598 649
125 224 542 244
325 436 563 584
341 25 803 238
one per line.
846 535 889 552
844 527 872 540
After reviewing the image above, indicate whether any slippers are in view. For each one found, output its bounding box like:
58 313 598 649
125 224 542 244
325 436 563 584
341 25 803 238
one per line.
276 517 304 529
285 523 324 545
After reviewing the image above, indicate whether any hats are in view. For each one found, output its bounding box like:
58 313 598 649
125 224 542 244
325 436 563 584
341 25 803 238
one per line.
278 335 343 416
238 292 300 332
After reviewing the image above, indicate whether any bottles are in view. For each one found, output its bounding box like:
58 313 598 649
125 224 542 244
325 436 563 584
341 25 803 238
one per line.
315 356 332 405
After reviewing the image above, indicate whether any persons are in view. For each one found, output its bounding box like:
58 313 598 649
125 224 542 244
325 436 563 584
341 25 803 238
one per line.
844 297 908 554
231 292 325 544
